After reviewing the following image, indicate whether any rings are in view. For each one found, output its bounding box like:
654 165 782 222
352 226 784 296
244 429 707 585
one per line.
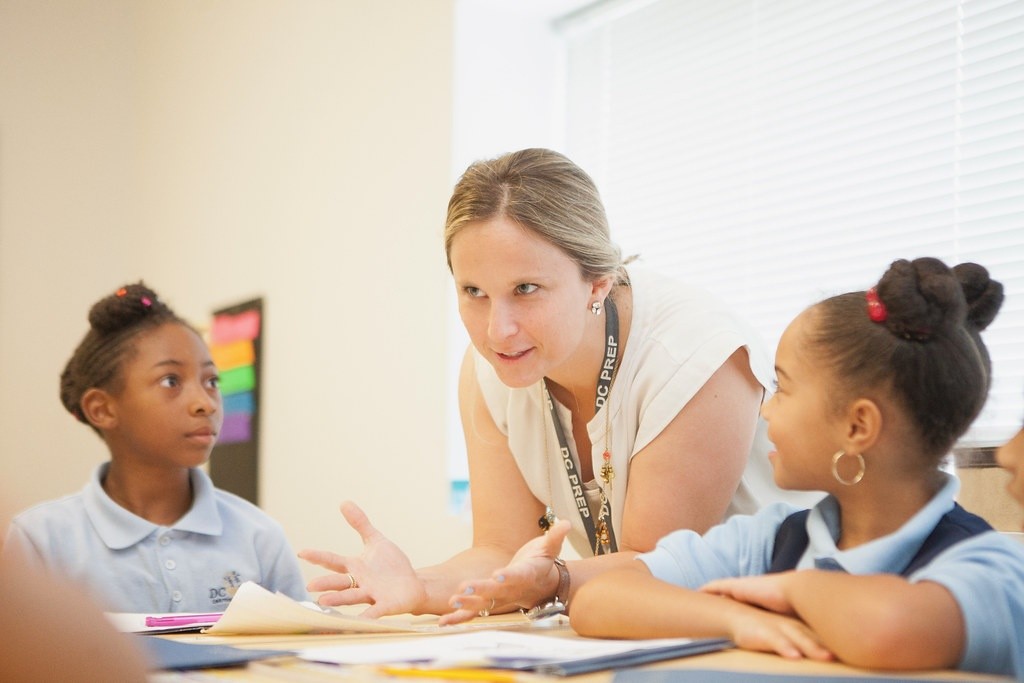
479 609 489 617
297 148 835 627
346 572 356 589
489 598 496 610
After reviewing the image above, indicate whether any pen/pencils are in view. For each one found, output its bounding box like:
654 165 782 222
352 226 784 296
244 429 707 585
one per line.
145 613 224 627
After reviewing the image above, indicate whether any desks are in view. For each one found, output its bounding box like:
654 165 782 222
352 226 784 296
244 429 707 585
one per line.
111 618 1020 683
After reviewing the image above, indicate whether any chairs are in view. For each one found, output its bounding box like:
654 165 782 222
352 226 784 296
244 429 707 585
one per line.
955 445 1023 530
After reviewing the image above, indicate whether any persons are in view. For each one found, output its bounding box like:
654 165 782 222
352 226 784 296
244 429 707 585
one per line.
1 515 152 683
9 281 311 614
996 426 1024 537
570 256 1023 680
